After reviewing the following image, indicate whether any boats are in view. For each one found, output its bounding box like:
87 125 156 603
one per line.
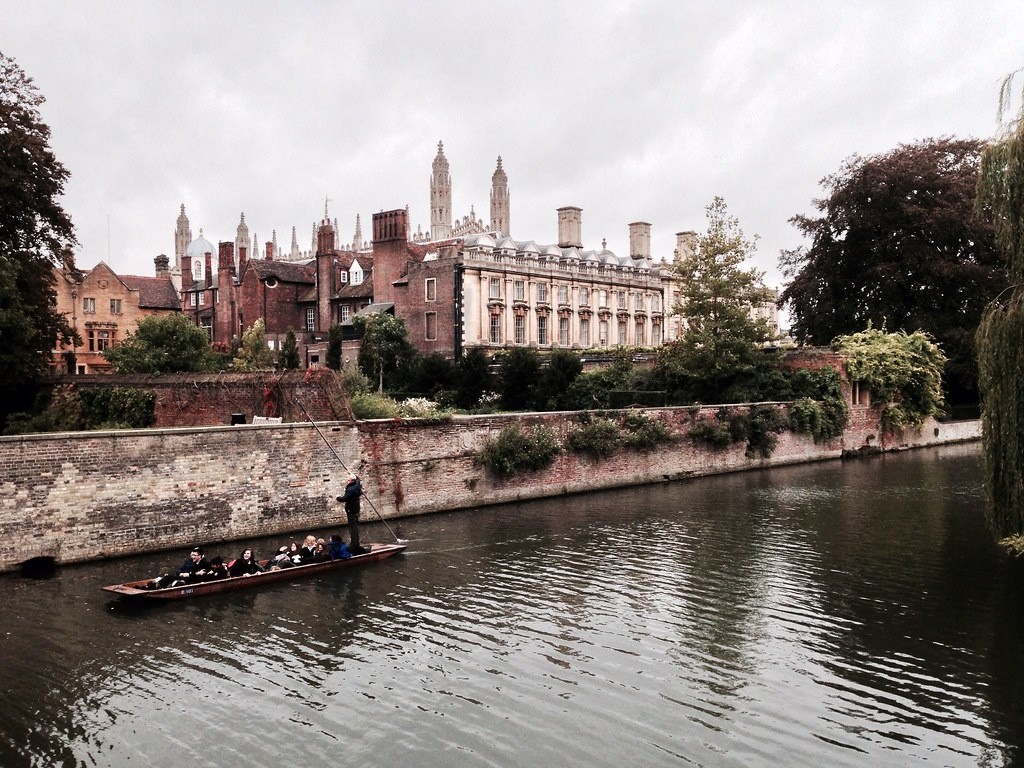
100 543 408 598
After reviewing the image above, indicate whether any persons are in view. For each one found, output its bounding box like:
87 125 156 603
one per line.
335 475 362 553
134 532 343 590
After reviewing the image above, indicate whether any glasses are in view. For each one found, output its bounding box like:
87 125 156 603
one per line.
192 555 199 556
